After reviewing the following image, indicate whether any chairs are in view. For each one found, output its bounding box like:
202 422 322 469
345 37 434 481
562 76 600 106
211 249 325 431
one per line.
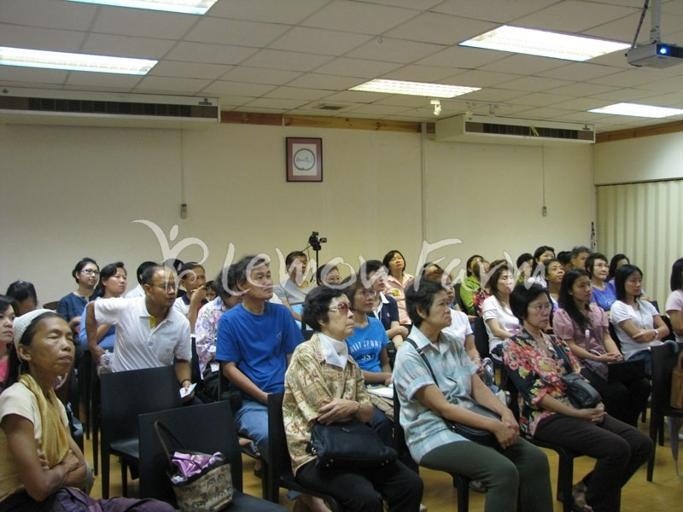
0 291 682 512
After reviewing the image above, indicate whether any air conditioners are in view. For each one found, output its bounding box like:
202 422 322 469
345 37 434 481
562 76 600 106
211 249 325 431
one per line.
0 84 222 132
433 111 597 149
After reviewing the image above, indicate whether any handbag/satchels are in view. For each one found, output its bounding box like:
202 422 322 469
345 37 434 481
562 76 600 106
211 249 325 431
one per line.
153 418 234 512
449 405 501 446
561 372 600 408
194 357 241 411
669 351 682 409
305 417 398 472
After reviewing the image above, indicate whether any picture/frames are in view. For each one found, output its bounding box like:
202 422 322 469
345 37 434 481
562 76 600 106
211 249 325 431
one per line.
285 137 323 183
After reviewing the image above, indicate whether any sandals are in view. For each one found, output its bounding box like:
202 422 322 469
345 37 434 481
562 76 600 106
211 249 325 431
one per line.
572 482 594 511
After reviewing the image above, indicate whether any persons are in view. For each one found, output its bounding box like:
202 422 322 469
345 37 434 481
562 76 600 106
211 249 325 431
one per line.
0 246 683 512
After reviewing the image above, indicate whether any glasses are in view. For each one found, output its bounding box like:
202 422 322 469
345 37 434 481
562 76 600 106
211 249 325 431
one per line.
79 269 99 275
328 302 348 313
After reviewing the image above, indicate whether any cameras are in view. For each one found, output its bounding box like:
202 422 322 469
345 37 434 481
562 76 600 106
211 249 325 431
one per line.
309 232 327 242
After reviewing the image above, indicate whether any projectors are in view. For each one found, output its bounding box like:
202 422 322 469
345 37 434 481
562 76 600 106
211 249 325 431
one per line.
627 43 683 69
